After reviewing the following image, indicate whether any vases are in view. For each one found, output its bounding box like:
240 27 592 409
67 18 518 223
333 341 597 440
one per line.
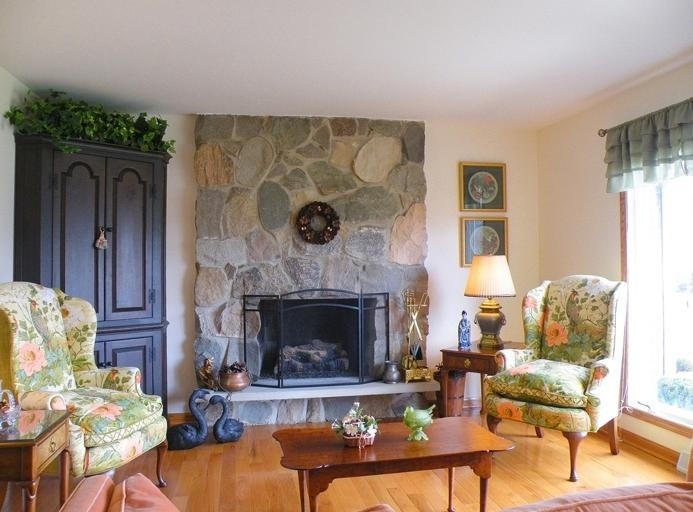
220 372 251 393
382 358 401 385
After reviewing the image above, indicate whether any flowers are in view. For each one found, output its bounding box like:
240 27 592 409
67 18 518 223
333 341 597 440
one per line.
221 361 247 374
332 400 380 438
295 198 342 246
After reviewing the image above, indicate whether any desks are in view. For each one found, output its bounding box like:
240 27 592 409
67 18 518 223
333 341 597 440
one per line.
0 402 77 512
435 337 547 445
268 412 518 512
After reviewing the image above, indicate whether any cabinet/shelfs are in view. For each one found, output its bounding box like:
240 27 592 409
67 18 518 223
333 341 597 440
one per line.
9 131 170 429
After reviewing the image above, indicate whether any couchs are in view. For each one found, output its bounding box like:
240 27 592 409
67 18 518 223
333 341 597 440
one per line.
56 444 693 512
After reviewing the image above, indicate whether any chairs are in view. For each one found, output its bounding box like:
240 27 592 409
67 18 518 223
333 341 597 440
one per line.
0 275 173 491
482 269 630 486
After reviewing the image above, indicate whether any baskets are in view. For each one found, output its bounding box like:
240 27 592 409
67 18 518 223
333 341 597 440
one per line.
343 435 374 448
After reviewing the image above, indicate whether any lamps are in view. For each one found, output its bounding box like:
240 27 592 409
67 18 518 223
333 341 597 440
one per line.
466 252 518 350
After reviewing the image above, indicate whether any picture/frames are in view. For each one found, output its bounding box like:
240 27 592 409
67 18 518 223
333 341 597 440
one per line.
458 160 508 214
459 216 509 270
